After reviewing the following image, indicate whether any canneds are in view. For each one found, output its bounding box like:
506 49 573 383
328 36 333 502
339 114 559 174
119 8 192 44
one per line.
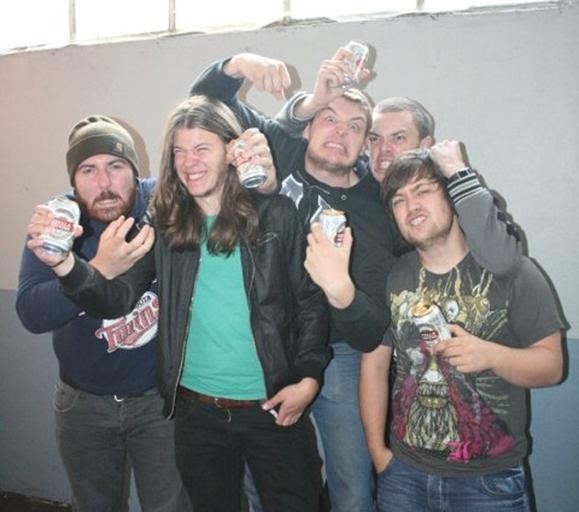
320 210 344 245
40 198 79 256
339 40 369 87
413 302 454 355
234 141 267 190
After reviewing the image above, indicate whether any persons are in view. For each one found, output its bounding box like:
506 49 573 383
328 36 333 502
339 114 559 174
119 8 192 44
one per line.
272 45 525 510
16 113 280 511
26 93 336 510
356 147 571 512
188 52 400 510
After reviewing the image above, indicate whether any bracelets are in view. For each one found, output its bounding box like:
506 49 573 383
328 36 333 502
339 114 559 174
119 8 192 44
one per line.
447 166 475 186
48 253 71 269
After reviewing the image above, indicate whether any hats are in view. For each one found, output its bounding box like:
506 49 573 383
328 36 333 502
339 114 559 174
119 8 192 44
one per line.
64 114 140 182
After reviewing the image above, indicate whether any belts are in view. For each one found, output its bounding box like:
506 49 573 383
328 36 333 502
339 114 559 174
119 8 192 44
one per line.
178 387 261 410
